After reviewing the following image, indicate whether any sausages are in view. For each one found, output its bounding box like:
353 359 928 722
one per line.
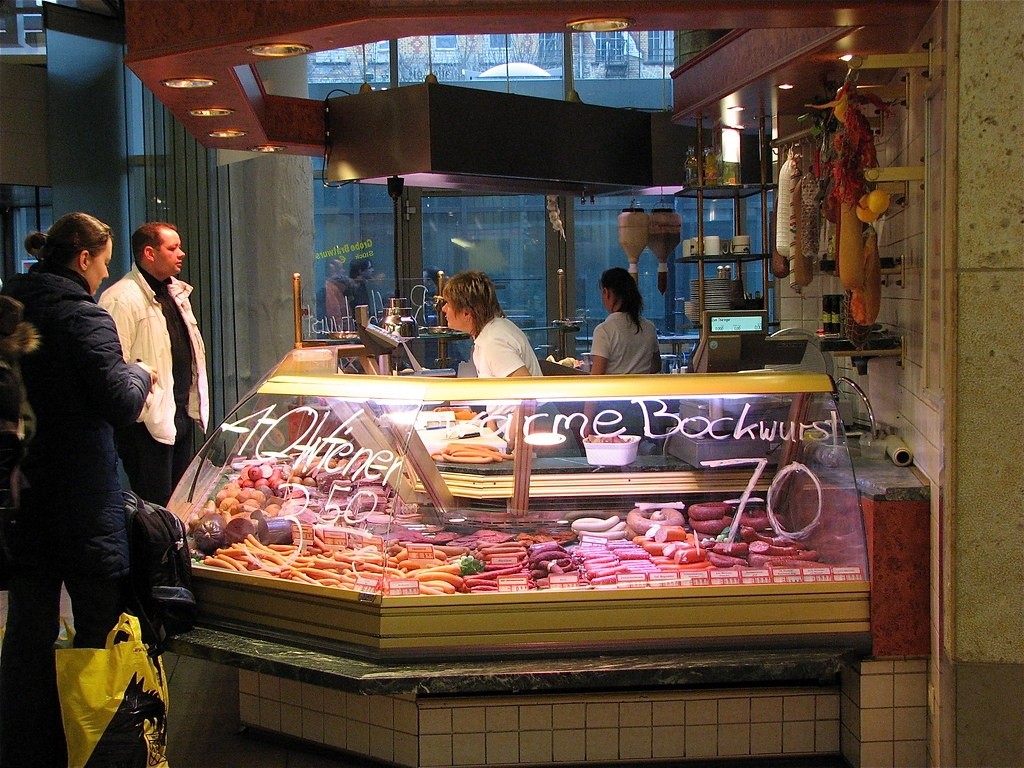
190 442 829 595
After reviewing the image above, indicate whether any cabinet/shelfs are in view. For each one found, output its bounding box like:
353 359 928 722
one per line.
820 49 931 355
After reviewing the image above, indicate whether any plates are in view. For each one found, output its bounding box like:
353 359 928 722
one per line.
684 279 731 323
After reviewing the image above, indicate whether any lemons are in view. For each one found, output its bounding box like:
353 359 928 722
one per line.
856 188 890 223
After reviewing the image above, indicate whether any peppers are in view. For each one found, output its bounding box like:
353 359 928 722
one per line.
801 82 893 206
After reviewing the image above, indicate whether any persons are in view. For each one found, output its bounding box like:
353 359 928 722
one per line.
316 257 388 339
422 266 543 378
0 212 163 768
96 222 209 509
590 267 662 375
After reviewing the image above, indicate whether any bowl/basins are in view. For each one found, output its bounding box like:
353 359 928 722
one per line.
583 435 642 465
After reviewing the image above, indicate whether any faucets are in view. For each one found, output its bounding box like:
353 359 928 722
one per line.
834 376 879 437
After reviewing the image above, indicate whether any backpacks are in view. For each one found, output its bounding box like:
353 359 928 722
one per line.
121 489 198 657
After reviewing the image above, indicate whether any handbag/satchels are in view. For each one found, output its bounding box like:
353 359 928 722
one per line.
0 615 77 652
56 612 169 768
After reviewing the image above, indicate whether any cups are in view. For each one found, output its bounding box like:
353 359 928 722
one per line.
730 236 750 255
721 239 731 255
704 236 729 255
683 239 691 257
691 237 704 255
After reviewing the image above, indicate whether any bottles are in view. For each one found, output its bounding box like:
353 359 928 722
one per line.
685 143 721 189
